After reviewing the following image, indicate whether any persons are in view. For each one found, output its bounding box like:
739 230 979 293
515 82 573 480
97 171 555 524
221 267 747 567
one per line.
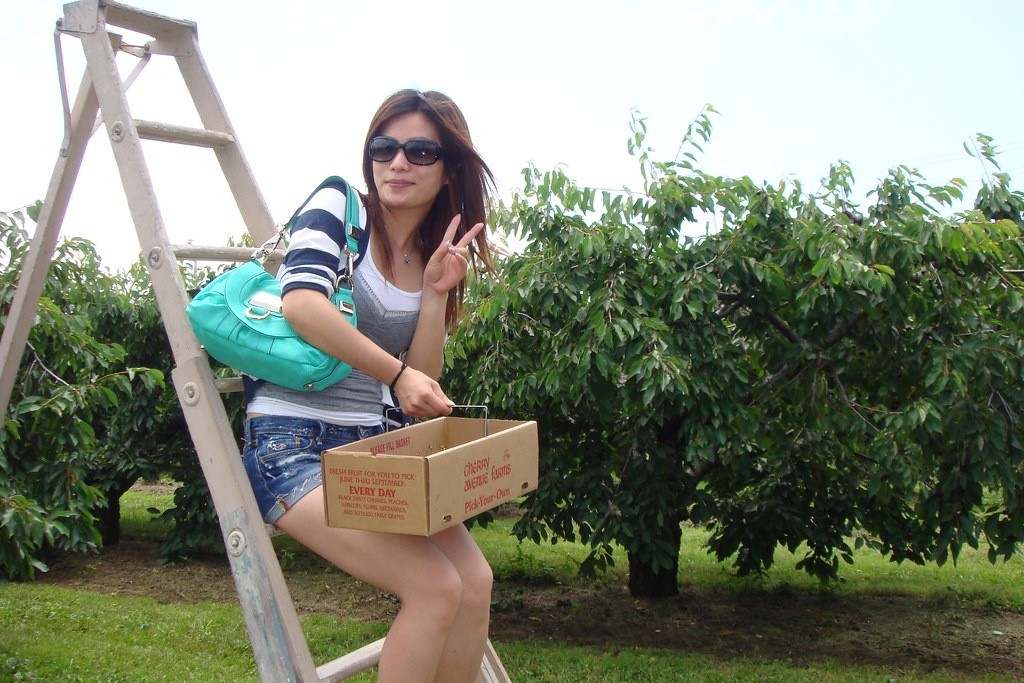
244 88 493 683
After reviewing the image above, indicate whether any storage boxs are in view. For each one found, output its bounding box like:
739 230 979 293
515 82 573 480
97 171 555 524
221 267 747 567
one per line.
321 416 540 537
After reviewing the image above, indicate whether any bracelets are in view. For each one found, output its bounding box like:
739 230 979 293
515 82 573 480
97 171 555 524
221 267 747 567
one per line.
389 361 407 389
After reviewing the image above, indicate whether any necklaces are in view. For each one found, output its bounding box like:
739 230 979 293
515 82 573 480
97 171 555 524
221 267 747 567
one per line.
386 228 418 263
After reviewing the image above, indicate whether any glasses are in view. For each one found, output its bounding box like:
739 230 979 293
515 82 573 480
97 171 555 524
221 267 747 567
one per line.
368 135 448 166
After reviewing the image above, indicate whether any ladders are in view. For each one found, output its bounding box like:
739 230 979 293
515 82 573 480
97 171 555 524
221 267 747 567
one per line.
1 0 537 683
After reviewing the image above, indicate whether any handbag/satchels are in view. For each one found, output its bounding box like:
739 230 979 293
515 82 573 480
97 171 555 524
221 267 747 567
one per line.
185 176 364 391
